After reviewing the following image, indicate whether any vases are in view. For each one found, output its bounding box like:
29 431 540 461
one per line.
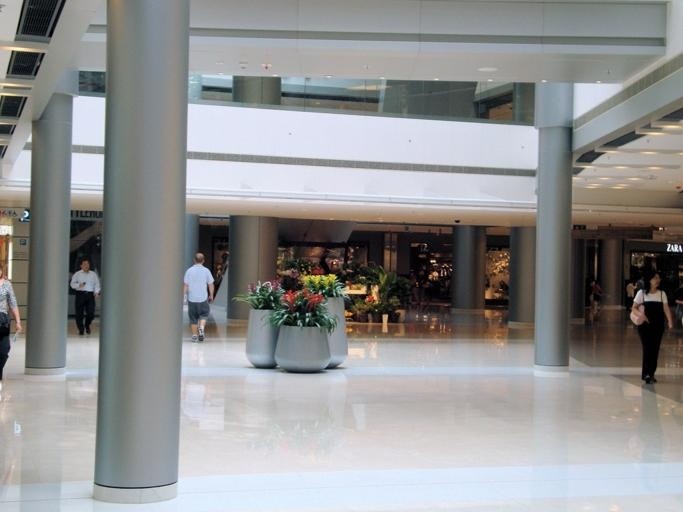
274 323 331 373
244 307 279 369
320 295 347 366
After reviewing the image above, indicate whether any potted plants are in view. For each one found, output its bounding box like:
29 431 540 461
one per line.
352 269 405 325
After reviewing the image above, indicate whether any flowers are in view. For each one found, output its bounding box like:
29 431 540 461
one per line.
231 280 286 311
297 273 343 298
267 287 339 332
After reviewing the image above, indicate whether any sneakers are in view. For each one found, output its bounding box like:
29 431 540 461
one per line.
192 334 197 342
198 328 204 341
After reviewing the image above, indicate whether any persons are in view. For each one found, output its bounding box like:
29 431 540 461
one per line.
409 264 426 304
69 261 101 335
583 261 682 320
0 264 22 381
182 253 215 343
631 270 672 383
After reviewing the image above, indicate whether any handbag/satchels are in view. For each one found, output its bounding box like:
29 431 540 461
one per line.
630 305 645 326
